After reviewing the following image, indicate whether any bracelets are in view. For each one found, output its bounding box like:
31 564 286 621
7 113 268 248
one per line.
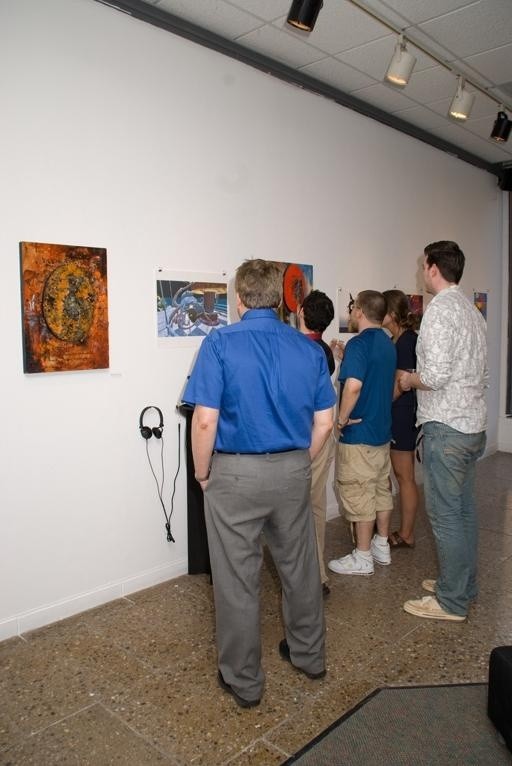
337 419 350 427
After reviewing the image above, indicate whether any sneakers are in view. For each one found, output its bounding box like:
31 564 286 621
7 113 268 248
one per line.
327 548 375 576
370 537 393 566
279 638 327 679
402 595 467 621
216 669 262 708
421 578 438 594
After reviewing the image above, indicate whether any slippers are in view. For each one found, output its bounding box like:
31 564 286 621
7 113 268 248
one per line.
388 530 415 549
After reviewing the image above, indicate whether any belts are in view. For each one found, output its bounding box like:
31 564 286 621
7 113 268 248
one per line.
215 447 299 457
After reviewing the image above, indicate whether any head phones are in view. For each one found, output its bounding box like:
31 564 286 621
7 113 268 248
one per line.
141 406 165 440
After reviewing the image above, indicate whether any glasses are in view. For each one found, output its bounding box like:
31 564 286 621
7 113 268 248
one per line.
350 304 360 310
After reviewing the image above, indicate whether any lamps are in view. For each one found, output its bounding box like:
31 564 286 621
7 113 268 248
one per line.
449 74 476 121
385 34 418 86
285 1 324 33
489 106 511 143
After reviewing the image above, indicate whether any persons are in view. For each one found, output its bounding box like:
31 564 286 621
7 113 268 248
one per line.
296 288 338 599
382 290 423 550
327 290 398 577
181 259 338 709
399 239 491 621
